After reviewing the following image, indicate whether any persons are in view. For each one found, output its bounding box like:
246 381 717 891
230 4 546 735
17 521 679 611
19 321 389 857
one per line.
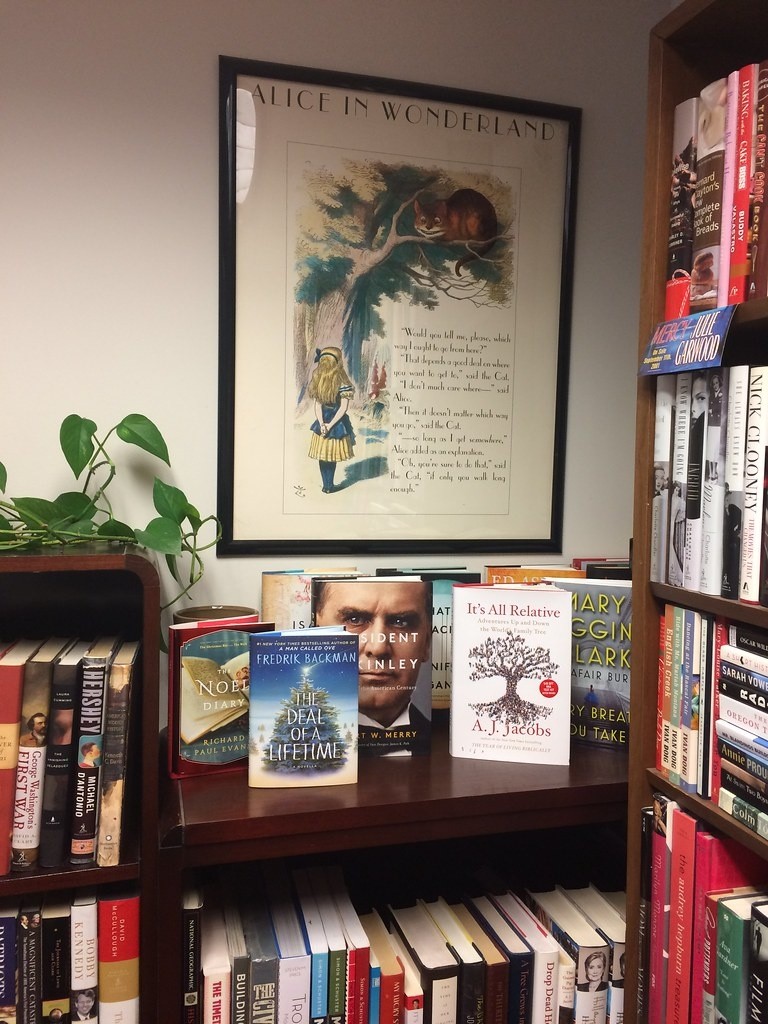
316 585 433 753
71 988 97 1020
578 954 608 990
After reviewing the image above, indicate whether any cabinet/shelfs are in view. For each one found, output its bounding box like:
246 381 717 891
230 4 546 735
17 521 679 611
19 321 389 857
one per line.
621 0 768 1024
158 724 629 1024
0 552 160 1024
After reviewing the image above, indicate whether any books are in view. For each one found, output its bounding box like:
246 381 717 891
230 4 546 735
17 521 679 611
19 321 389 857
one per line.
0 63 766 1024
247 623 359 788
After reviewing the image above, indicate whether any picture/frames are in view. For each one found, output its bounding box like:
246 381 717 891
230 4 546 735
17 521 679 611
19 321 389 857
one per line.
214 54 584 555
